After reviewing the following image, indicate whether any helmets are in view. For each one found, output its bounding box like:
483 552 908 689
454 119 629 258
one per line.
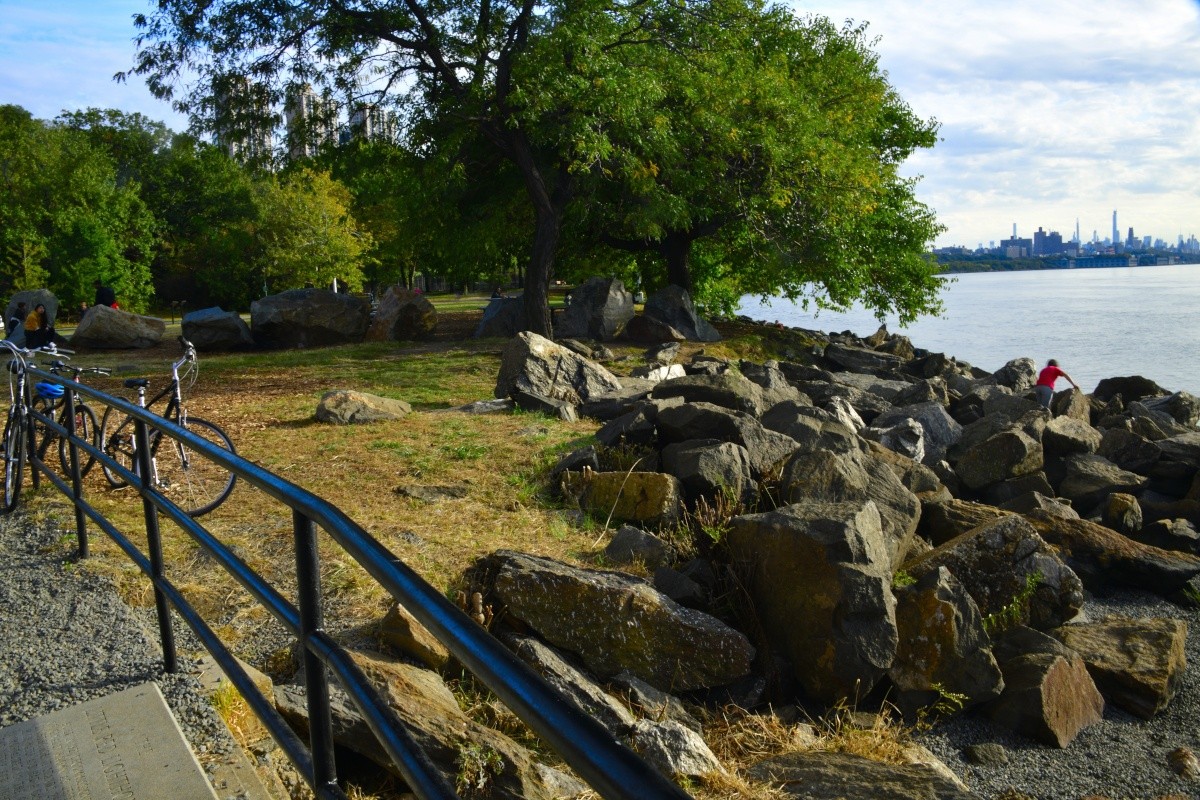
35 379 64 398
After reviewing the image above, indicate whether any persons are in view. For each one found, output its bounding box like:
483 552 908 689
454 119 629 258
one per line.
1036 359 1080 407
491 287 507 298
23 303 47 358
93 280 111 308
9 302 30 353
416 287 426 295
78 300 90 320
37 313 58 354
111 300 120 310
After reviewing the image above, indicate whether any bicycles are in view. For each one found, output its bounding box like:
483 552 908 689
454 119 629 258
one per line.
0 340 76 512
27 360 113 479
99 334 239 517
